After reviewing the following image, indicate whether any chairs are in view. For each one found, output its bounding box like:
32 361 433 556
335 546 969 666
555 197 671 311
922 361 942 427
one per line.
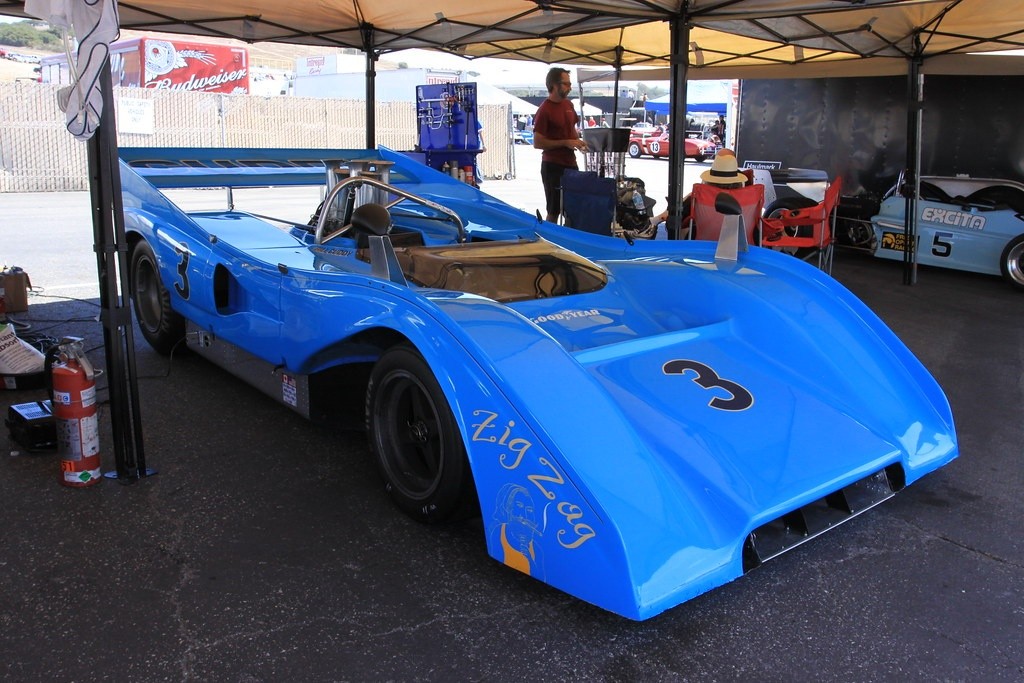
364 230 424 249
690 169 841 275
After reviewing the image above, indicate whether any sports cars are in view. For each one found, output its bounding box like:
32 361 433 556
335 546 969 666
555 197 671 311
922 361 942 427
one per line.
117 143 960 622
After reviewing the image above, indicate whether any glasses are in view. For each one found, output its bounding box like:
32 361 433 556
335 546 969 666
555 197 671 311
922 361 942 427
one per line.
559 82 571 87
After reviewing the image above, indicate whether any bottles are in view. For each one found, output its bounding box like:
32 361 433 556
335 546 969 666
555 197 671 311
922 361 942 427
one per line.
442 160 475 186
632 190 645 214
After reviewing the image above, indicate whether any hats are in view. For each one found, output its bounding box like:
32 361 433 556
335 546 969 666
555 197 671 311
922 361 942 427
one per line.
701 154 748 185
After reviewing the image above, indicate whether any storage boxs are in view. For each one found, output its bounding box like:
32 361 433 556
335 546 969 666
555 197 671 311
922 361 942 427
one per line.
0 272 32 314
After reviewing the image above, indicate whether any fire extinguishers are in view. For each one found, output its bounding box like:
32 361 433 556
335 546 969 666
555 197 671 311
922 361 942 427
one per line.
44 335 104 488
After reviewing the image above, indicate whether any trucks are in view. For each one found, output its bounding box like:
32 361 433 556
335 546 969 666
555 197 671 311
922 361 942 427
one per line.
33 36 250 94
280 68 466 102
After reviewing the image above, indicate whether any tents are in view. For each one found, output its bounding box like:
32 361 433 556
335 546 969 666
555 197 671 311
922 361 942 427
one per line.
645 83 728 128
570 98 602 128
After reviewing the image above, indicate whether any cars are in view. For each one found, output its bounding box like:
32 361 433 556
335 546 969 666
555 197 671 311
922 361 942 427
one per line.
629 133 716 162
0 48 41 64
762 172 1024 291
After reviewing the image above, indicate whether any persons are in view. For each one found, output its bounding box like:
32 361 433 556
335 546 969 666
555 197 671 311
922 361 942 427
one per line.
574 115 615 138
655 122 670 134
709 114 726 148
620 88 649 102
632 148 749 240
513 113 533 144
533 67 591 225
